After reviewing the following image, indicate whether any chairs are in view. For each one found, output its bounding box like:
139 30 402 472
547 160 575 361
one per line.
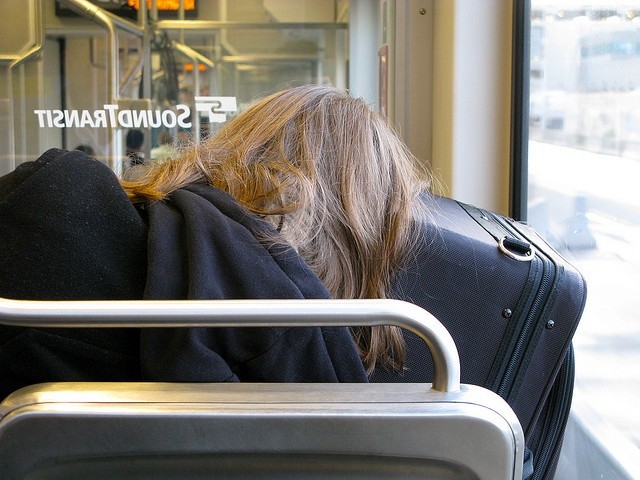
0 382 525 480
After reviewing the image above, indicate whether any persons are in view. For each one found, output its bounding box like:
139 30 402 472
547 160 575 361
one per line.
150 131 180 164
122 128 146 181
2 83 431 403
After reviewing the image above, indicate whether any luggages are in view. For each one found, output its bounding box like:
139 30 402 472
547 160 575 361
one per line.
388 193 587 477
